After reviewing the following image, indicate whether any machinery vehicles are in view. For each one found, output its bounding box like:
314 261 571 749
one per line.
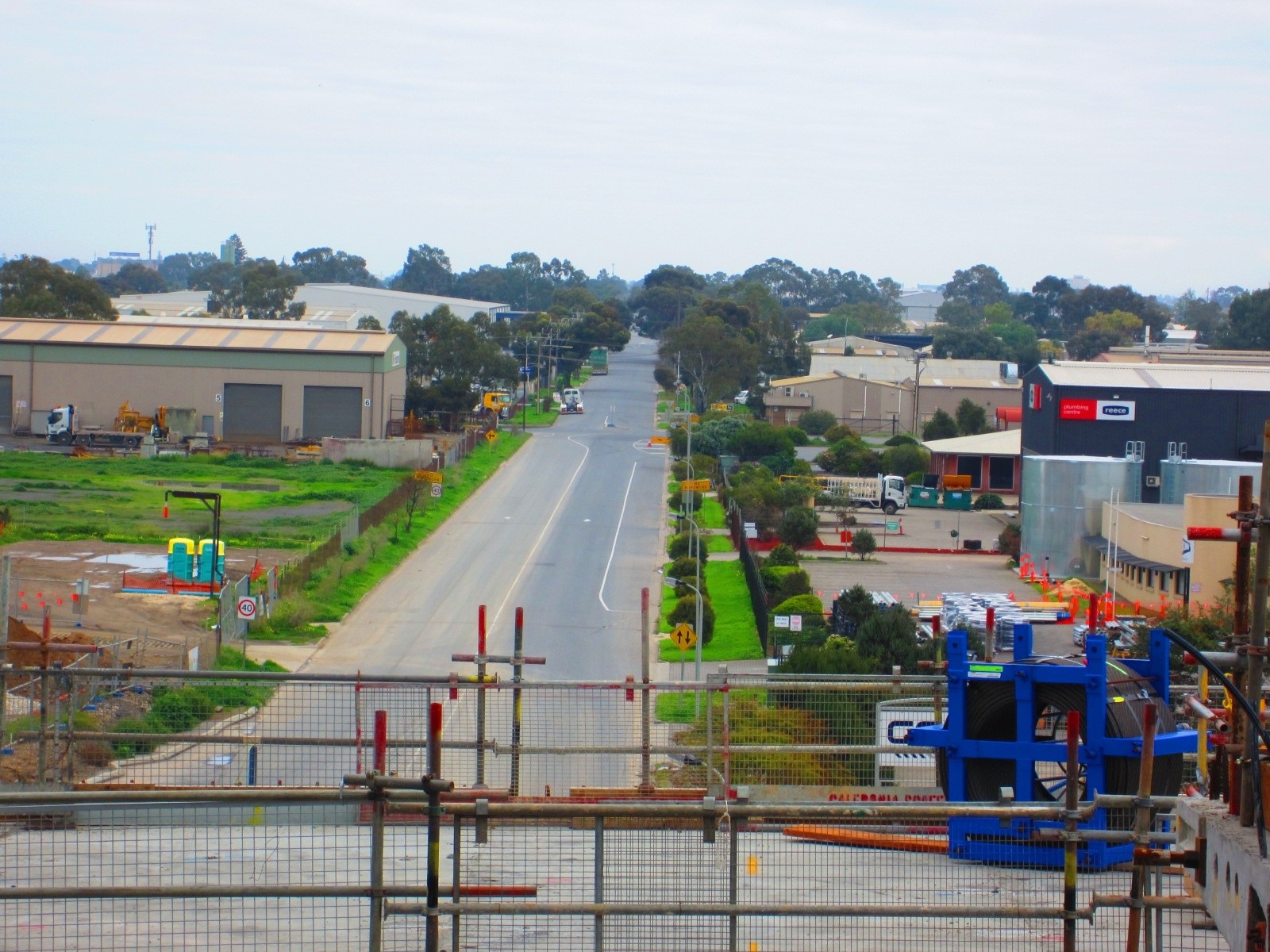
116 400 169 446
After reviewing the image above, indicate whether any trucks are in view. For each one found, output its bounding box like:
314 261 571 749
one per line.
473 389 513 421
559 387 585 415
45 403 147 450
590 346 609 375
815 474 909 515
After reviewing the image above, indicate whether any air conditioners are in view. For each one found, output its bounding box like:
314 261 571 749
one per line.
1146 476 1159 487
799 393 808 397
785 386 794 396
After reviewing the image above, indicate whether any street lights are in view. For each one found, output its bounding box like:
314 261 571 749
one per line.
666 510 702 682
666 454 694 560
670 421 690 518
663 572 705 723
